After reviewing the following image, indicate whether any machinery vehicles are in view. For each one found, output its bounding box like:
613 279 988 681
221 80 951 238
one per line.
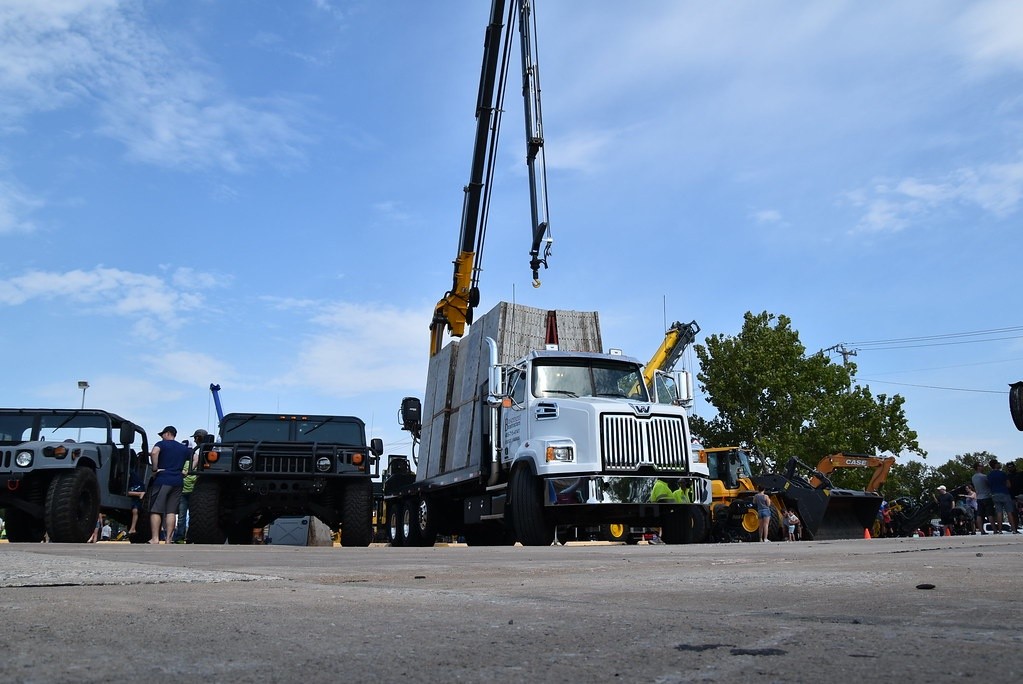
706 447 895 543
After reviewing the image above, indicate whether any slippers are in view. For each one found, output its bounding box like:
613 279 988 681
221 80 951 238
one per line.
142 540 151 545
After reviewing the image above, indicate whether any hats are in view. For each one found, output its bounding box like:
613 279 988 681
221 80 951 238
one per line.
937 485 946 490
158 426 177 437
104 520 110 524
190 429 207 437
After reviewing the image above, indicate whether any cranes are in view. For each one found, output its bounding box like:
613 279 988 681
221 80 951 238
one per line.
382 0 712 546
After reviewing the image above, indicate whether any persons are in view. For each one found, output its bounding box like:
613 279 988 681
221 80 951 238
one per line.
174 459 198 543
101 520 112 541
753 484 772 542
883 506 893 533
958 460 1023 535
146 426 192 544
932 486 955 535
191 429 208 469
781 509 801 541
126 469 146 534
650 479 693 503
93 514 104 543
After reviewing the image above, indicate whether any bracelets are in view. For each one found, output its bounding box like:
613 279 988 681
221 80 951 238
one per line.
152 470 157 472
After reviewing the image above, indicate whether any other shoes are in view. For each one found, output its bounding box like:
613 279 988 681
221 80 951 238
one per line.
764 539 771 542
760 540 763 543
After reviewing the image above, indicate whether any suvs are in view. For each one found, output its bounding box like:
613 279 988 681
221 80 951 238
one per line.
0 408 156 544
188 412 384 547
